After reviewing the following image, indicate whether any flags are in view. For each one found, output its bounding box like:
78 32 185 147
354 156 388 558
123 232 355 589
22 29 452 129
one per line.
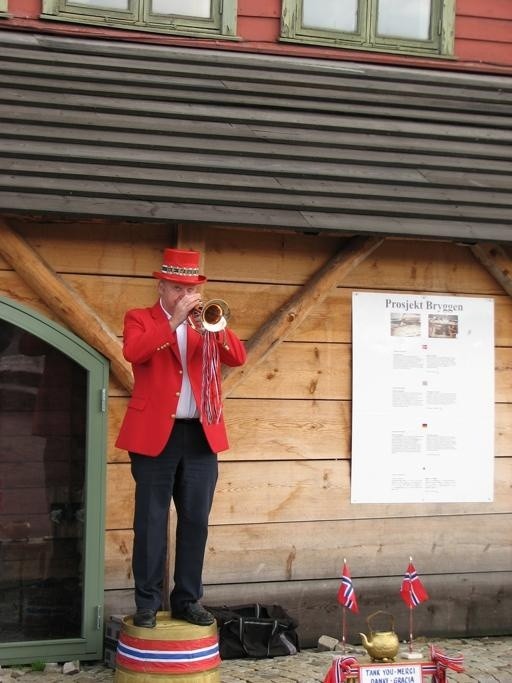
337 563 358 615
400 563 429 609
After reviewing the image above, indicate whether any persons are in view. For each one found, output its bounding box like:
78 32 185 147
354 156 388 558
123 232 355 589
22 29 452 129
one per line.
114 249 247 629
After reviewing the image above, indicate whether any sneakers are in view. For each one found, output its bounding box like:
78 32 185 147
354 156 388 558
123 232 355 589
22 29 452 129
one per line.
171 600 214 624
132 609 158 628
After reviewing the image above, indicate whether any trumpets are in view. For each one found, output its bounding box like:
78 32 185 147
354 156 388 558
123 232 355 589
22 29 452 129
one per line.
188 299 231 332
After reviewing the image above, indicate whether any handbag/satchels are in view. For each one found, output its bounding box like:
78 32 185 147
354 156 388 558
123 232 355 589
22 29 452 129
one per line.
206 603 298 659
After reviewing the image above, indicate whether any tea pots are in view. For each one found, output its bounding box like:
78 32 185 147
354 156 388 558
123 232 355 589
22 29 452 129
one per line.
358 610 400 659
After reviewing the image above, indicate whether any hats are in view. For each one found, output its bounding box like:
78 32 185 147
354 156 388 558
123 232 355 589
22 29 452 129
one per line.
153 249 208 285
